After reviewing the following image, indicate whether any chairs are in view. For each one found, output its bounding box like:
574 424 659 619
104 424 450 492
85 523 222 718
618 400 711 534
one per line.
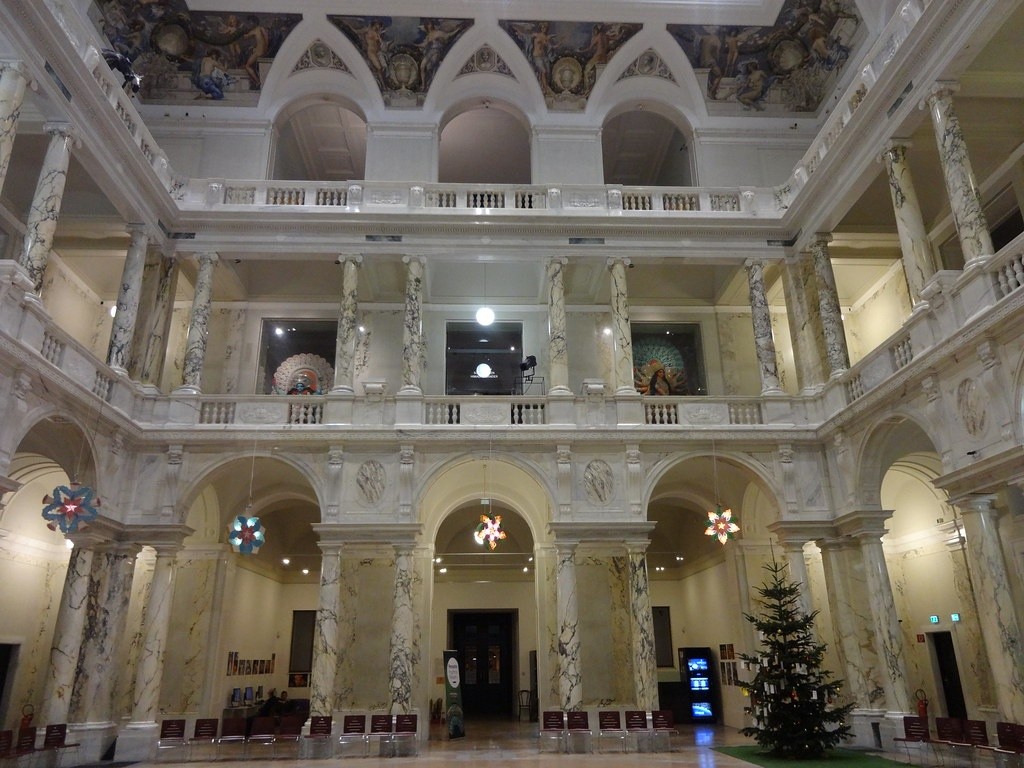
539 711 564 753
277 715 306 761
893 716 939 768
219 717 247 754
19 727 43 768
188 718 219 761
518 690 531 722
922 717 972 768
625 711 655 753
338 715 369 758
962 719 996 768
1014 724 1024 768
367 715 396 757
157 718 186 763
0 729 19 768
566 711 594 754
247 716 277 757
652 710 682 752
304 716 333 760
390 714 419 756
43 723 81 768
598 710 628 756
994 722 1018 768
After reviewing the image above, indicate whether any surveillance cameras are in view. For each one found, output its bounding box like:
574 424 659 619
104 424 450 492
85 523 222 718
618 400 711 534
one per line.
520 355 536 371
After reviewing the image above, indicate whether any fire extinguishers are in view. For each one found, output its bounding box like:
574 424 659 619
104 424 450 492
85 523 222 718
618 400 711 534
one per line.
916 689 929 717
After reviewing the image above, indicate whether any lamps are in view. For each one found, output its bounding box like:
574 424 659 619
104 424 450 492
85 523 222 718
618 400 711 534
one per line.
703 440 740 547
41 398 98 536
475 263 495 327
474 431 508 551
476 363 491 379
228 430 266 556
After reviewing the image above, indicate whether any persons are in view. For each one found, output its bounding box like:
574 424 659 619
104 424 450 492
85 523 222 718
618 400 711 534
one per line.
286 372 321 396
634 358 688 396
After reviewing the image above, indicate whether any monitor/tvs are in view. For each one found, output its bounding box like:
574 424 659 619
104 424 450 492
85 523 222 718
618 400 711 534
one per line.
245 687 253 700
233 688 241 702
688 659 708 671
258 685 263 698
692 702 712 716
690 678 709 690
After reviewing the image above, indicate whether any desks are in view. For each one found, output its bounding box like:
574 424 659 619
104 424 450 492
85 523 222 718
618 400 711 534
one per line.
224 705 259 719
